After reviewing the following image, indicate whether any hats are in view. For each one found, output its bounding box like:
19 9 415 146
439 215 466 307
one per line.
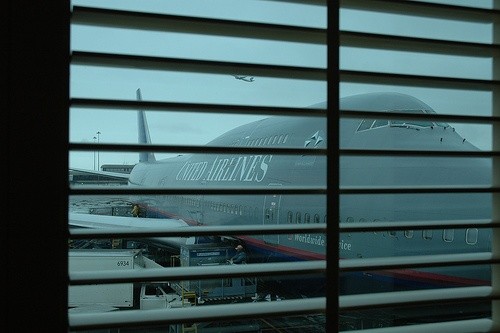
235 245 243 250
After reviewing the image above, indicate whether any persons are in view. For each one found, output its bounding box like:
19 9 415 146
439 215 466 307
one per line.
131 204 139 217
229 245 247 264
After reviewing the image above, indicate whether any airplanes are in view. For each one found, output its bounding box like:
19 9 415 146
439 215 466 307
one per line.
70 88 494 317
